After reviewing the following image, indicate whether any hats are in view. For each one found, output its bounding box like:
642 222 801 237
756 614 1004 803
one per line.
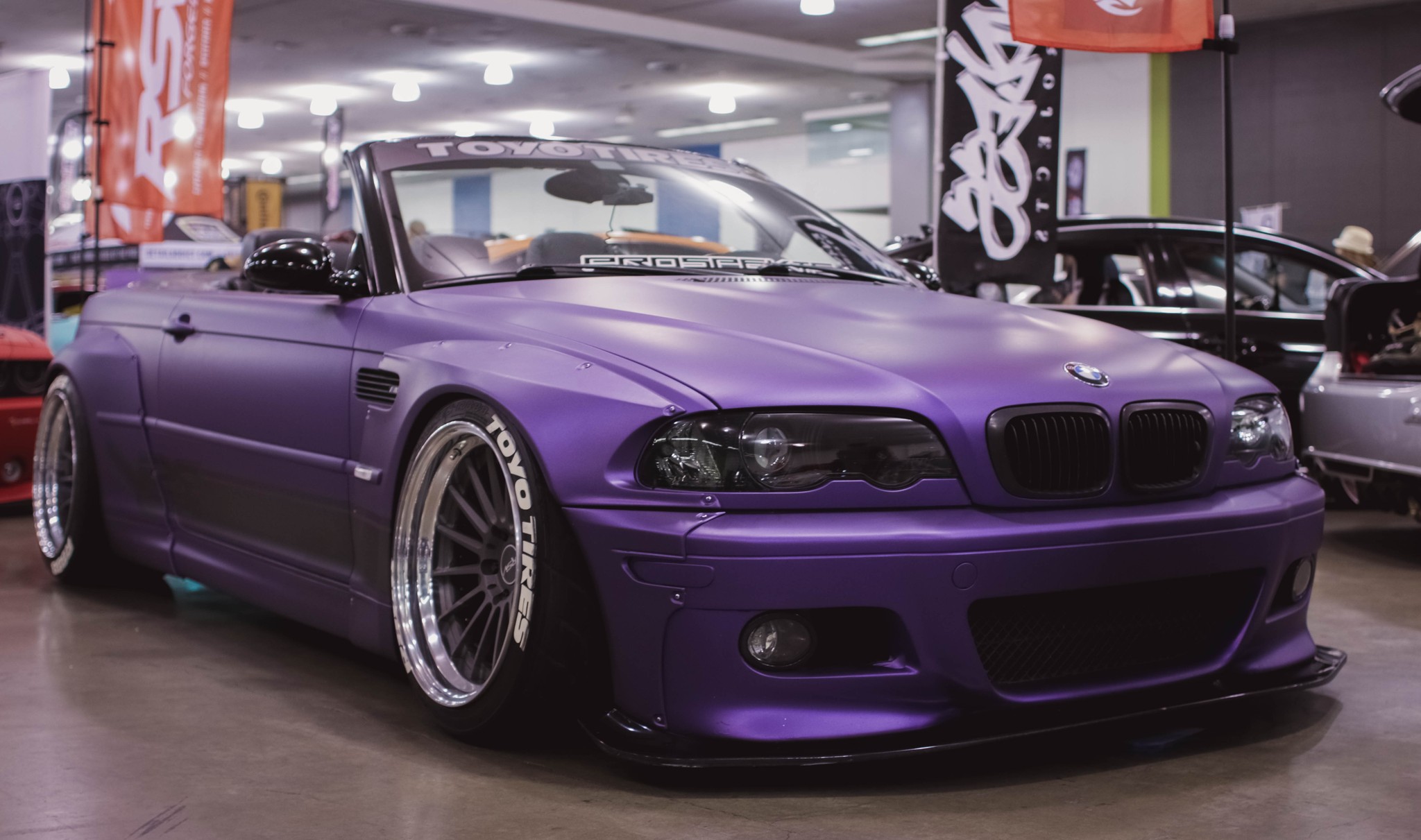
1333 226 1373 256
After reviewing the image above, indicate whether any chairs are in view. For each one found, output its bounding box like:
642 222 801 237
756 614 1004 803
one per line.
525 230 611 266
404 234 491 277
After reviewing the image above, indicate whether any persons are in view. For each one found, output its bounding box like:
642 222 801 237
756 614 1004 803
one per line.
1240 225 1380 310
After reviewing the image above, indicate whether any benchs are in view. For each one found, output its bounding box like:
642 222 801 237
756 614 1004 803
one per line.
240 228 352 271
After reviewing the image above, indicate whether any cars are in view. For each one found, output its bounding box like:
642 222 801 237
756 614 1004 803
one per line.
35 140 1348 786
881 215 1394 418
0 325 56 506
1299 64 1421 515
